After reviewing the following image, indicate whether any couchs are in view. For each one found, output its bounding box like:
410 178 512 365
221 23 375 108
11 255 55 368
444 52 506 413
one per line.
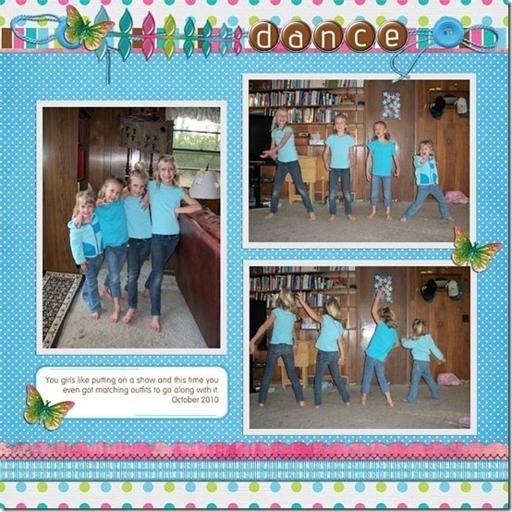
176 203 220 349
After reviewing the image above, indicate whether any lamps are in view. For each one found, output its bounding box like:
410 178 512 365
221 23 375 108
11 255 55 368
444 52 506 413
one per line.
188 168 220 209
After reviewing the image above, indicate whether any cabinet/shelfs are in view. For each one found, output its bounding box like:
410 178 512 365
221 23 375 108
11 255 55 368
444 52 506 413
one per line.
249 87 366 147
250 270 357 332
250 347 267 393
250 161 275 209
161 199 220 274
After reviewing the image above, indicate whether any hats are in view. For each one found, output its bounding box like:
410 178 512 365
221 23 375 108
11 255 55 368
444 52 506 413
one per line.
448 281 458 297
421 279 437 301
429 96 445 117
457 97 467 114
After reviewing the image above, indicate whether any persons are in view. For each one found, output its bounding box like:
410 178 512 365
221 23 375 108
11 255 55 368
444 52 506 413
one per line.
259 109 319 221
68 155 201 334
249 291 304 410
360 288 398 409
366 120 400 222
323 113 354 222
401 320 447 404
295 291 352 407
400 141 454 223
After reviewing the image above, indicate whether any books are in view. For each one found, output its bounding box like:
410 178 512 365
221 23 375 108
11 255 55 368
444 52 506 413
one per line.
250 266 356 308
248 79 364 125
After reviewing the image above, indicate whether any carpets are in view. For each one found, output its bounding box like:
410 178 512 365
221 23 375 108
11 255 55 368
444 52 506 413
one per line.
43 270 85 348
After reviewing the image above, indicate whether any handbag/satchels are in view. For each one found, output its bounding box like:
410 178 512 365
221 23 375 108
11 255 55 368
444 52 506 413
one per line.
446 190 468 204
437 373 461 386
